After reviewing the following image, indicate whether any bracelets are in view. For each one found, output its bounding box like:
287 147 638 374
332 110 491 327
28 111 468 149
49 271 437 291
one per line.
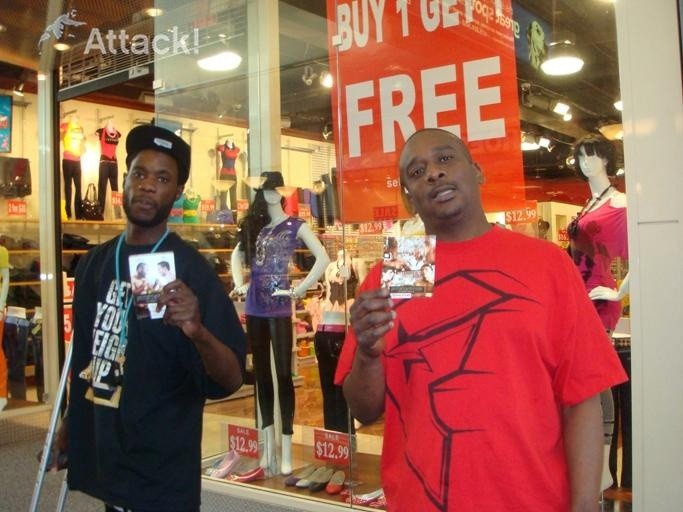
291 288 305 301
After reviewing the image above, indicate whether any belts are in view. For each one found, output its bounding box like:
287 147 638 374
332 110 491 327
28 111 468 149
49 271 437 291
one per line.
317 325 345 333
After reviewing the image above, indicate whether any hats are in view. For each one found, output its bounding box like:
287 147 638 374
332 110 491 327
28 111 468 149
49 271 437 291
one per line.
126 125 190 171
253 172 284 191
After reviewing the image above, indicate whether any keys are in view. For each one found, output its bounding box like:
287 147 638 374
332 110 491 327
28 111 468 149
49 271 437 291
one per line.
115 354 126 377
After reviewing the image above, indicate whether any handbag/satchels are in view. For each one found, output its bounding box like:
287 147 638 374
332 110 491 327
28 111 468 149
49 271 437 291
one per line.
81 199 104 220
206 209 235 224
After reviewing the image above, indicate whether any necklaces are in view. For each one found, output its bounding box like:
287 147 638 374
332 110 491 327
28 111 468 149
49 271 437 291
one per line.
254 214 281 266
567 185 616 239
335 262 345 277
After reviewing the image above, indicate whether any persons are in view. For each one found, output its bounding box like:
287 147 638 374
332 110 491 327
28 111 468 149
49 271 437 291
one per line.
181 187 203 225
329 129 628 512
565 129 631 495
129 262 152 321
379 237 434 299
38 122 246 512
0 244 14 411
30 306 44 402
93 122 122 221
3 304 29 402
155 261 174 317
316 251 366 454
213 137 240 226
55 121 87 221
166 191 184 223
228 170 330 476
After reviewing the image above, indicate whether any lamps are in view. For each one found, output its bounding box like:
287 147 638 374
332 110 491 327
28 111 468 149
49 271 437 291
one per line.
538 0 584 76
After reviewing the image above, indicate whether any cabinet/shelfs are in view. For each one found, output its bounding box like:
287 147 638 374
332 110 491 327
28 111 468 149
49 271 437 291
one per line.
0 214 323 373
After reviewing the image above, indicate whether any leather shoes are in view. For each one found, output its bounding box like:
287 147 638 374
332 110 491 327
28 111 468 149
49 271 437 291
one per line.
207 449 264 483
346 488 386 508
285 466 345 494
